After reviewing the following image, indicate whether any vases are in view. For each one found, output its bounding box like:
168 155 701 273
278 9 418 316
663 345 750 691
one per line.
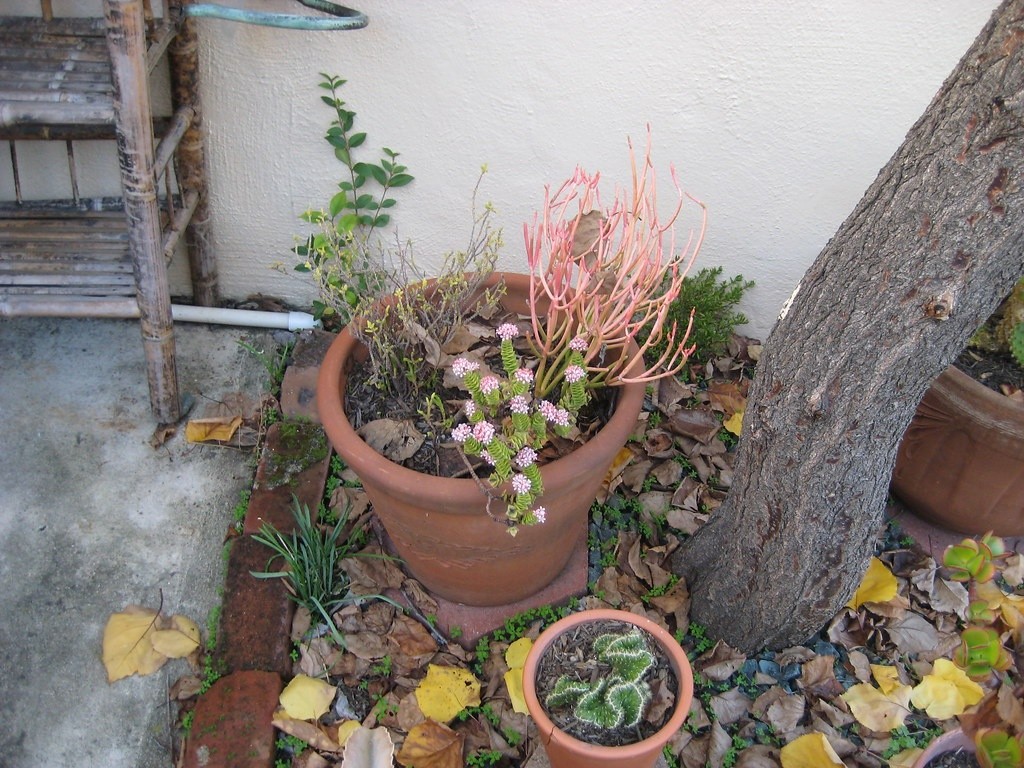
317 272 646 607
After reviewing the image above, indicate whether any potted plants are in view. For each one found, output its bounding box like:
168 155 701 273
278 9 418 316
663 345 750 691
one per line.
522 609 694 768
890 275 1024 537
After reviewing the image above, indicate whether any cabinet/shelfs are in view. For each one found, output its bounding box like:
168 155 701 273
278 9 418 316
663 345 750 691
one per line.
0 0 218 423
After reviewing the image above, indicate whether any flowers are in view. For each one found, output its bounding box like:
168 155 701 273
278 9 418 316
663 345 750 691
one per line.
268 124 706 538
